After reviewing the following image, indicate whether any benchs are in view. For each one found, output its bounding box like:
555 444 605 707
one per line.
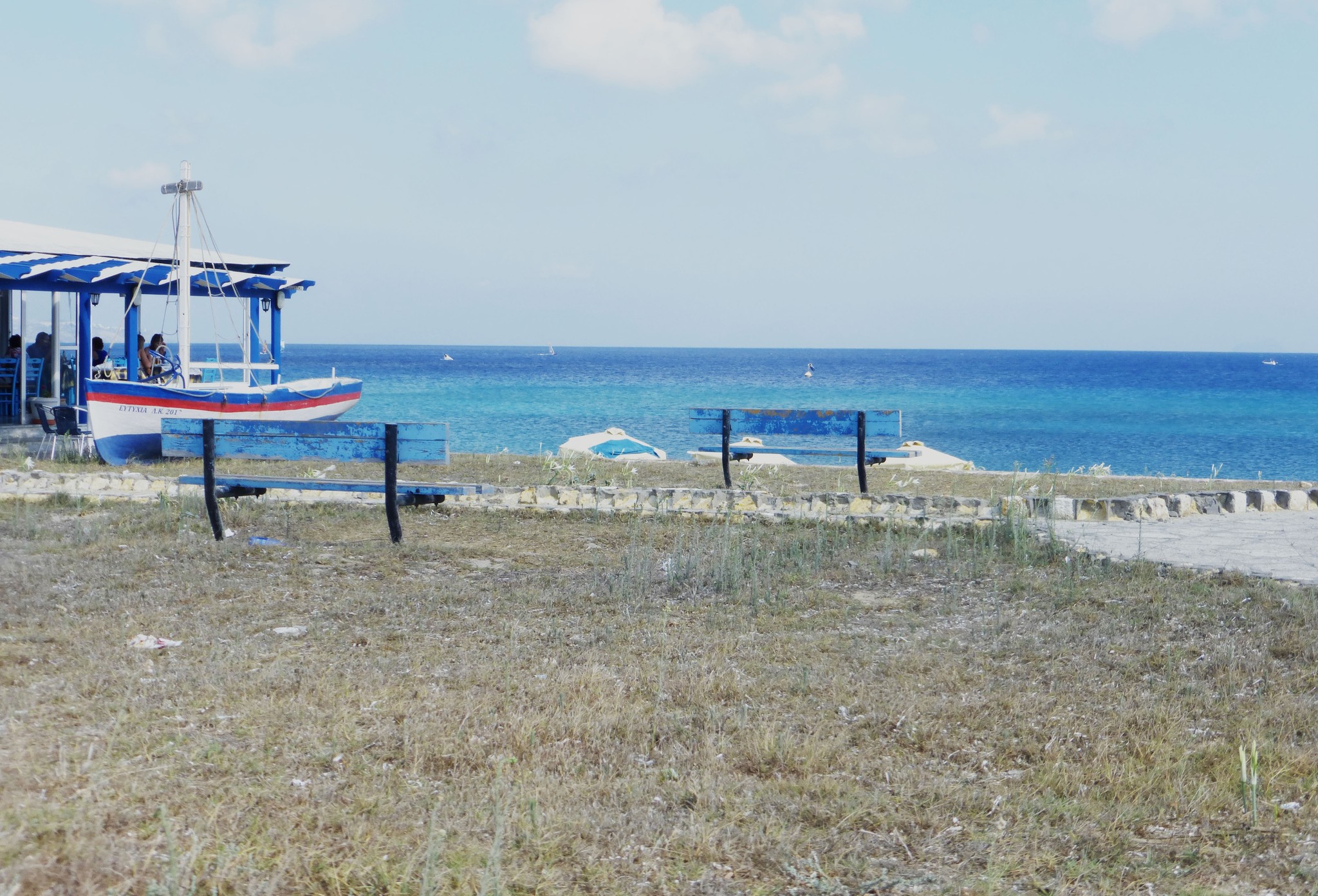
687 406 922 493
159 415 497 542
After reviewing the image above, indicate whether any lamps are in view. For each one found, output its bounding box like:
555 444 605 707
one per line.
89 292 101 307
260 298 271 312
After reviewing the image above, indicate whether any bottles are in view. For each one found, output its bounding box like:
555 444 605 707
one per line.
248 536 285 548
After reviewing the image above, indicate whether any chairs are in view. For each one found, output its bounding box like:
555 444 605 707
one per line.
49 405 98 460
2 355 218 426
35 403 80 463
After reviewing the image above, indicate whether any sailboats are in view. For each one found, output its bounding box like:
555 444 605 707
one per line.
82 151 365 466
259 339 268 354
280 340 285 354
548 342 557 355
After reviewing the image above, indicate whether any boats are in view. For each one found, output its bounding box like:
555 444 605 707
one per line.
1262 358 1278 365
804 363 815 378
444 354 454 360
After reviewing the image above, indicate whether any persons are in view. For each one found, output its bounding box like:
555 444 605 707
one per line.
126 334 152 378
145 334 167 376
1 335 36 414
91 337 111 379
26 332 51 397
